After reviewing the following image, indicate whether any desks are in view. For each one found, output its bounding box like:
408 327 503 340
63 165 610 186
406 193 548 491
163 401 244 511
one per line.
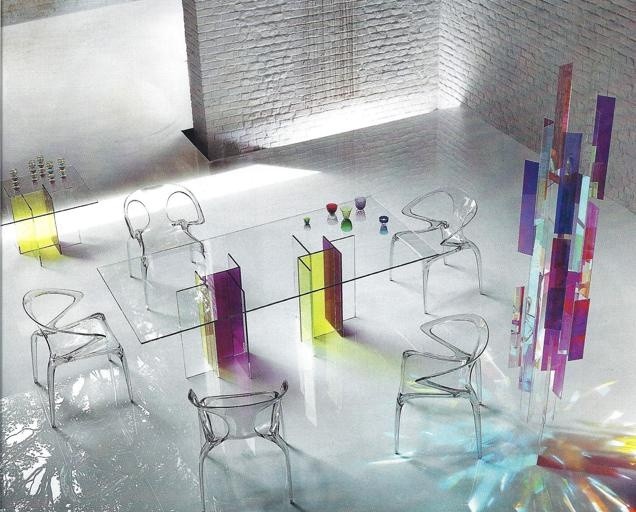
0 164 98 265
94 194 439 381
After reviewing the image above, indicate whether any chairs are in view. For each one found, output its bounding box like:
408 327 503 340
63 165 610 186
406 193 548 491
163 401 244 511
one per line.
387 183 490 459
186 379 292 510
21 287 134 430
123 181 209 309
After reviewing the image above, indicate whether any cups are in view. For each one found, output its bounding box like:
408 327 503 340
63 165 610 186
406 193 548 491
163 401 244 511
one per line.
354 197 365 210
303 216 310 225
304 224 310 231
327 214 337 225
378 216 388 223
326 203 336 216
356 210 365 222
340 205 352 219
379 224 387 234
340 219 351 232
